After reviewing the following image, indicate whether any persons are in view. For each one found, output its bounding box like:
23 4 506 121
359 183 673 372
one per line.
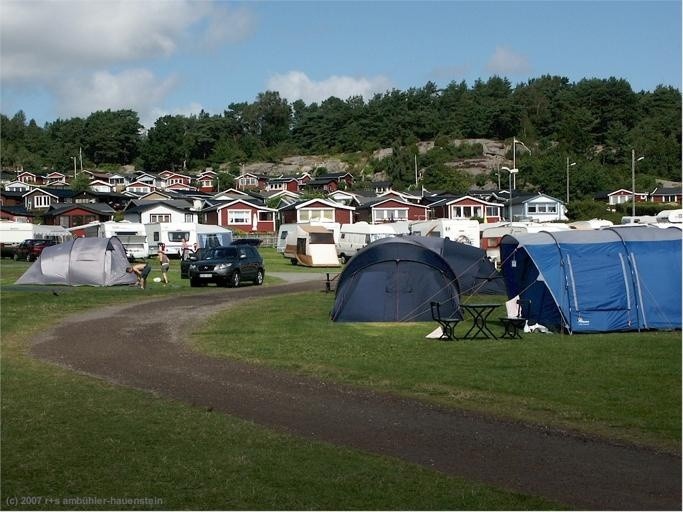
180 238 198 260
157 251 170 287
126 264 151 290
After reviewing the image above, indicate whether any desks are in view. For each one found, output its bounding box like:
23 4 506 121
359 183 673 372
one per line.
458 303 501 341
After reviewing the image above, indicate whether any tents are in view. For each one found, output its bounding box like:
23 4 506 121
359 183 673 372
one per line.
12 236 136 288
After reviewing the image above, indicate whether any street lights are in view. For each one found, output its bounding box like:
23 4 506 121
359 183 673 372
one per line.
501 166 518 222
631 148 644 216
566 156 576 203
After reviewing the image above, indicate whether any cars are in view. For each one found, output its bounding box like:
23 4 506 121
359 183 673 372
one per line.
180 239 263 280
13 239 53 262
189 244 264 287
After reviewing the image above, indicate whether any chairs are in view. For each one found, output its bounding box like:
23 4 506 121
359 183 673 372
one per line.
497 299 532 339
429 301 459 341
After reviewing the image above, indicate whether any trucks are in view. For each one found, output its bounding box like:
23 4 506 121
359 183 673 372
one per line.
276 219 481 269
64 219 234 258
